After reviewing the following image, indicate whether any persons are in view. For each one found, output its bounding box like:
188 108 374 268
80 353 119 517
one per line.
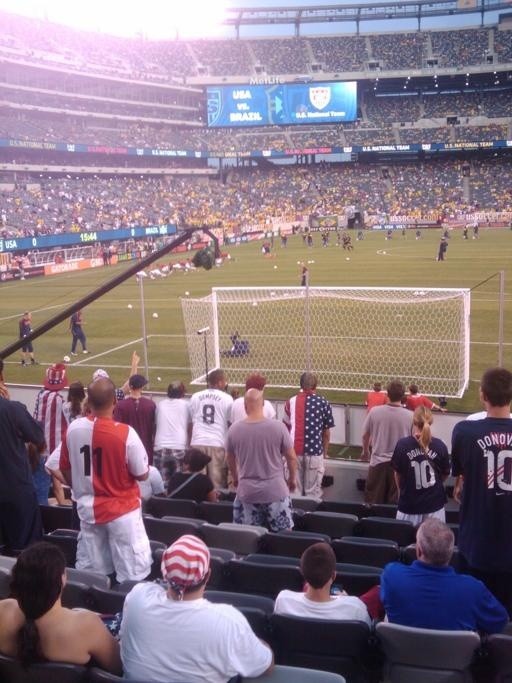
167 449 221 503
152 380 193 480
25 441 72 514
0 24 512 280
83 351 140 415
452 411 512 504
361 381 413 504
405 385 448 412
59 376 154 586
272 542 383 633
218 331 250 357
0 542 122 673
451 368 512 606
112 374 156 465
32 363 69 452
117 535 275 683
137 465 164 501
18 311 38 367
379 518 508 637
62 381 86 426
188 370 233 489
390 405 450 527
365 382 388 412
225 388 298 533
69 312 91 356
281 372 335 498
44 397 91 531
0 361 44 549
301 264 308 284
229 374 277 427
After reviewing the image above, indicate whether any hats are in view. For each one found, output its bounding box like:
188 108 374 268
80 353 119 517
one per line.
44 364 68 391
160 533 211 601
128 374 148 389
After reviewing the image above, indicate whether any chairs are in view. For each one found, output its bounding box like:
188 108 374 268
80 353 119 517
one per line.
37 504 79 531
315 500 370 516
84 584 128 617
403 539 465 568
332 533 399 567
66 568 111 589
413 519 459 542
41 531 84 566
201 520 268 552
359 514 416 546
482 631 512 677
240 606 267 640
240 663 344 682
2 656 88 683
216 484 237 503
259 529 329 556
144 514 203 542
226 553 305 599
146 491 198 517
290 492 319 510
147 545 235 588
444 506 463 523
58 582 87 613
374 619 481 683
86 666 127 680
335 562 383 597
266 613 374 683
303 508 359 534
200 588 275 611
0 570 12 596
368 498 401 519
147 539 168 550
200 499 239 519
0 555 18 570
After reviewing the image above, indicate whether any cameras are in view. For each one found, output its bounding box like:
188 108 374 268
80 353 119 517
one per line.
190 244 216 271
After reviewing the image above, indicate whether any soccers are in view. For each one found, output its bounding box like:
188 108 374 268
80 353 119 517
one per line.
312 260 315 264
414 291 418 296
63 356 70 362
274 265 277 269
297 261 300 265
153 313 158 319
284 293 288 297
421 293 425 297
383 251 386 255
128 304 132 309
308 261 312 264
346 257 350 261
253 303 257 306
185 292 189 295
271 292 276 297
